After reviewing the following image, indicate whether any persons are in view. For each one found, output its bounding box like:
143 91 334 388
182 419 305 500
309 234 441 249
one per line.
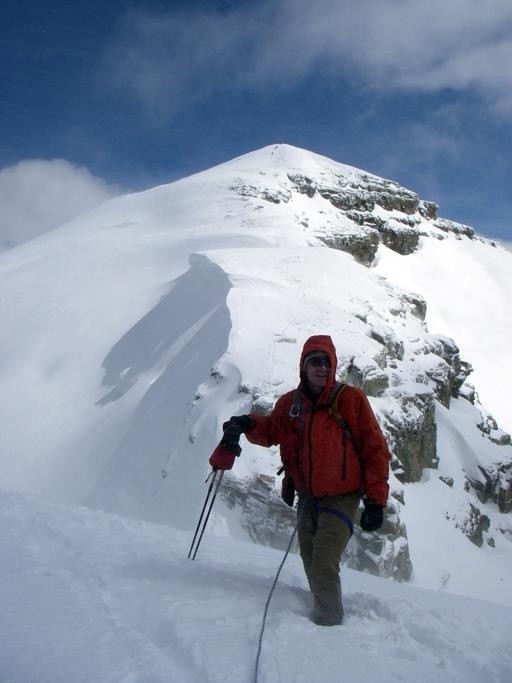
223 335 389 626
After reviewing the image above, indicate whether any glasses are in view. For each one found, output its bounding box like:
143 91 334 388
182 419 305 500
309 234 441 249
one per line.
307 357 332 368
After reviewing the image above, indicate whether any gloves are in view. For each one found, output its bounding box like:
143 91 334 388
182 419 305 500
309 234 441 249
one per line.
223 415 252 436
360 498 383 531
